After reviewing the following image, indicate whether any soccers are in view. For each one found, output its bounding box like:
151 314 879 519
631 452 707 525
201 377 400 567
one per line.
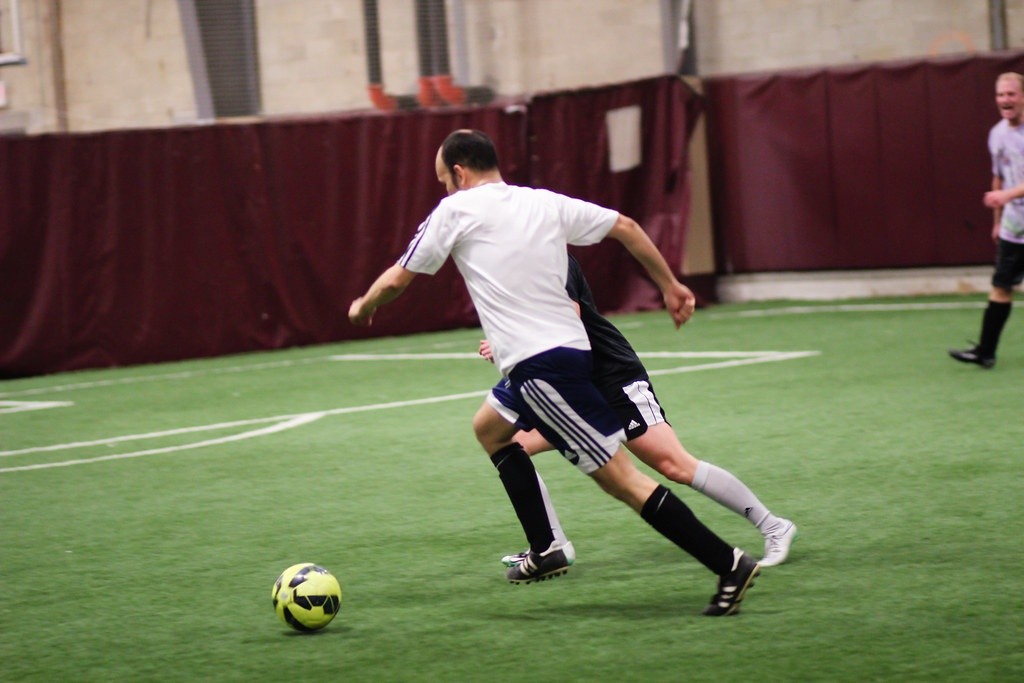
269 562 344 633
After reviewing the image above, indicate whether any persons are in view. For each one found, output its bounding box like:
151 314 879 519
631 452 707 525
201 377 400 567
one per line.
349 125 762 614
946 70 1023 368
477 251 797 571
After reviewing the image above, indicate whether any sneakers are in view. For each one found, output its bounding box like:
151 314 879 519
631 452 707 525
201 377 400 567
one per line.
703 547 761 616
948 340 996 369
501 541 575 566
756 518 797 567
506 551 568 585
